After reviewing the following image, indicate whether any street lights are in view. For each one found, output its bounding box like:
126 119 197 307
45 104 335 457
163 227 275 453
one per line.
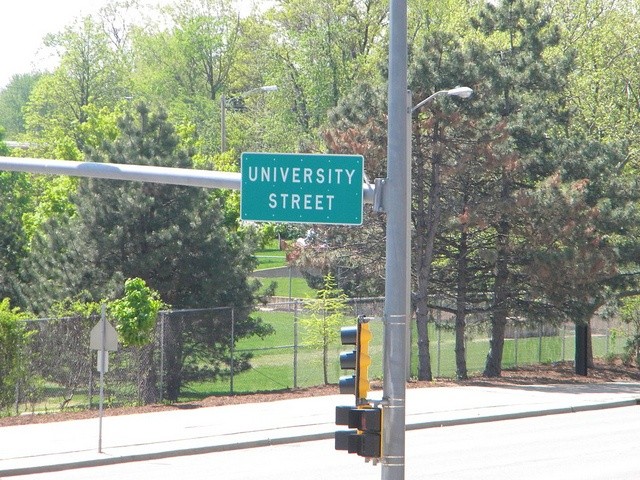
407 86 472 381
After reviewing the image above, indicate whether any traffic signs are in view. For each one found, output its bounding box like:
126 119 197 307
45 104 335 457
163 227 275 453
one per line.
240 152 364 226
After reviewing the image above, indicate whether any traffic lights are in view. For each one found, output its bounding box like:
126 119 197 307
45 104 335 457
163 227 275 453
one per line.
335 406 358 450
339 315 372 399
349 407 383 458
